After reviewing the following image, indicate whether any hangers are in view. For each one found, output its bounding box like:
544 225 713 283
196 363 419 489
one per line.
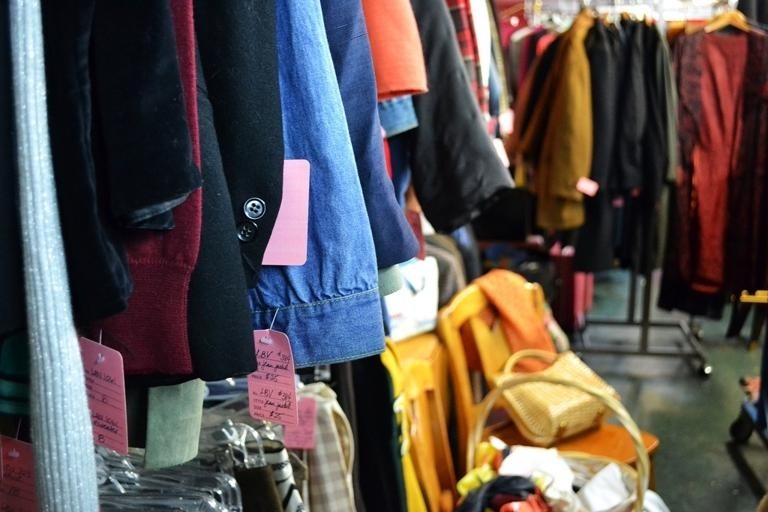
533 0 767 48
75 396 266 512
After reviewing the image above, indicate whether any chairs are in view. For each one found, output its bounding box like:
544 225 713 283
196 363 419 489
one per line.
436 271 659 512
379 335 458 512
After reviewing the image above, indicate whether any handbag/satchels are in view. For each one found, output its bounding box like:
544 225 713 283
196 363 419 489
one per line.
498 348 622 448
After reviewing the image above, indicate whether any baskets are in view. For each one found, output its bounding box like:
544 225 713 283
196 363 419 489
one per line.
466 374 650 512
